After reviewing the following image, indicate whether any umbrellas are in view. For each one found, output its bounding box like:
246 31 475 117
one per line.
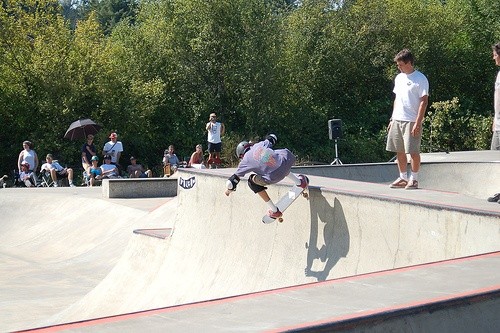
64 119 98 139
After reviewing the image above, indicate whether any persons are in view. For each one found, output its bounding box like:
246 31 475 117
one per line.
164 146 187 173
87 156 104 186
189 145 206 168
127 157 152 178
386 49 429 189
102 133 123 167
226 135 307 219
41 154 76 187
100 155 118 179
20 161 39 186
488 41 500 203
18 142 38 174
206 113 225 168
82 134 98 171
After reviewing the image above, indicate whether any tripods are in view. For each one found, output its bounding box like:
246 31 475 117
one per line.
330 139 343 165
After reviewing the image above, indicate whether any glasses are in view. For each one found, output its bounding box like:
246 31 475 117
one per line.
87 138 93 140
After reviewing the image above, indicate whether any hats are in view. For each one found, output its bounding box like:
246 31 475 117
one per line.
130 156 135 160
90 156 99 161
108 133 117 138
103 155 112 160
20 161 30 168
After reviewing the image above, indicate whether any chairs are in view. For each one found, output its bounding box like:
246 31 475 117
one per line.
13 160 68 187
83 171 102 186
163 163 171 177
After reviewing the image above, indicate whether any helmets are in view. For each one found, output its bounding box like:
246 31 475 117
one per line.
236 141 254 159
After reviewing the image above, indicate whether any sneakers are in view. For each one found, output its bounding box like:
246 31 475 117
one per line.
389 176 408 188
296 175 306 188
267 207 281 218
405 179 418 190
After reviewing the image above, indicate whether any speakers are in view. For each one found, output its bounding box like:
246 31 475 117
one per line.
327 119 343 140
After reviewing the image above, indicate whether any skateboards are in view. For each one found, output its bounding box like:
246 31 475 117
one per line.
262 175 309 223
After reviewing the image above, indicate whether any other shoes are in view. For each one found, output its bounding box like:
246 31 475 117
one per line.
207 158 213 163
37 184 43 187
487 193 500 203
53 183 58 187
70 184 76 187
216 159 221 164
